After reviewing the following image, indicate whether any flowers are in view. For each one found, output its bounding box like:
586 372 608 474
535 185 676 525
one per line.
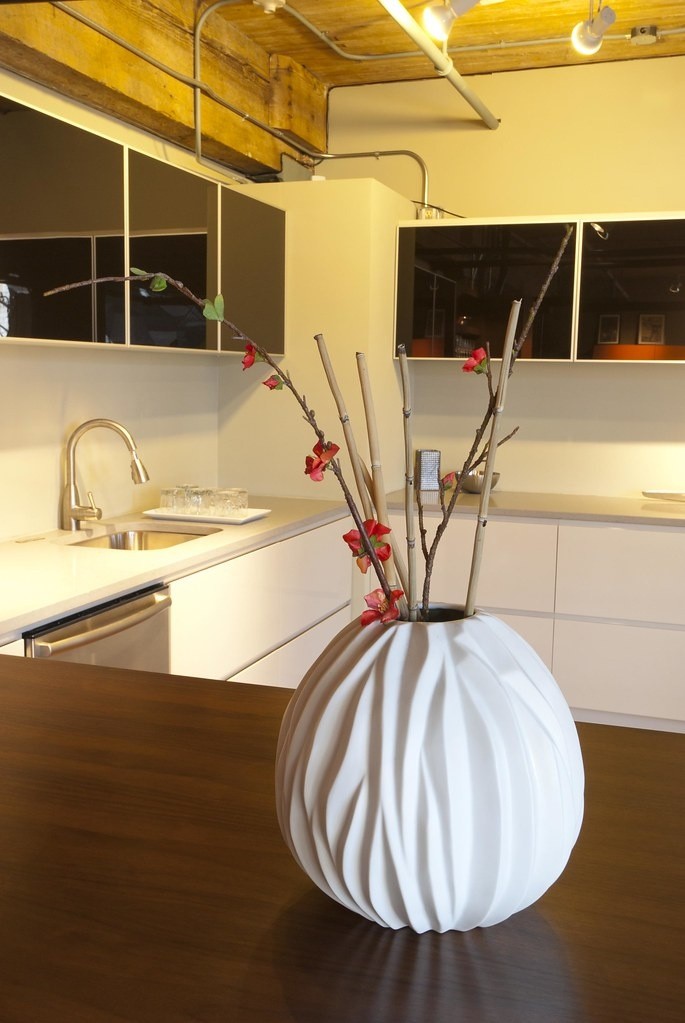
39 223 577 632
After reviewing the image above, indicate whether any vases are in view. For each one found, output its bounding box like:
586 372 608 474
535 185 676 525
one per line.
272 600 586 936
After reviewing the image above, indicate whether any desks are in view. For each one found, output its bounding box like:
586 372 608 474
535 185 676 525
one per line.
166 515 354 693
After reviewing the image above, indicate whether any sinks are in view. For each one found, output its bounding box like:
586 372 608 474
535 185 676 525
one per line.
61 523 226 553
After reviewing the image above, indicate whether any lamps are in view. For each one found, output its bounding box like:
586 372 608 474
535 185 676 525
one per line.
570 6 616 57
423 0 479 43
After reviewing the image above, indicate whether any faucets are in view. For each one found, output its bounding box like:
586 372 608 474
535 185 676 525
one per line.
57 418 151 532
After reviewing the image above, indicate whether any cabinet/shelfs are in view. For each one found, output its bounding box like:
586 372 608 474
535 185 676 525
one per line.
369 504 685 721
